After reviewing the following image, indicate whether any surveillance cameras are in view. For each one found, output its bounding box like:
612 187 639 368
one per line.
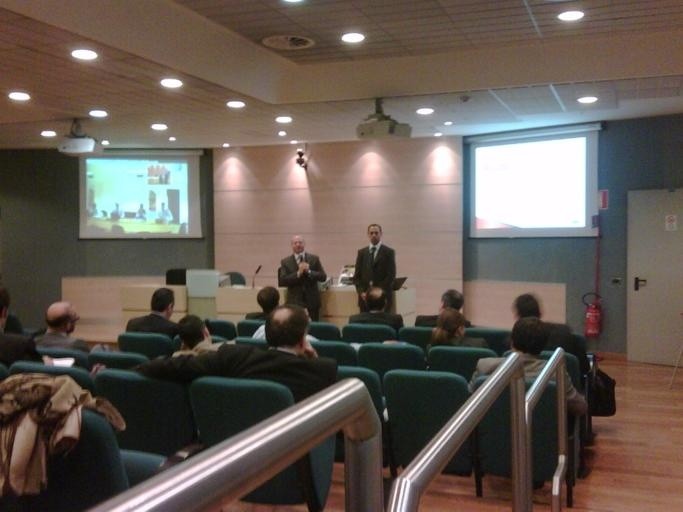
294 148 308 167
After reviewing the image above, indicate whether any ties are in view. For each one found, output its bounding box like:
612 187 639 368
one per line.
369 247 375 264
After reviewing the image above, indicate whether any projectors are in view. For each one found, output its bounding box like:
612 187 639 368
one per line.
57 136 104 156
357 115 411 140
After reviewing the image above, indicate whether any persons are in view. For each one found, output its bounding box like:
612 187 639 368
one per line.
86 200 186 235
0 283 606 512
278 235 326 322
351 224 396 313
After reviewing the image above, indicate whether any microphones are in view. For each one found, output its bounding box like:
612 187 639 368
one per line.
250 263 262 287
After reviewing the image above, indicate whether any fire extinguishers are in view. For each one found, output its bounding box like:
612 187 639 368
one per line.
582 292 604 339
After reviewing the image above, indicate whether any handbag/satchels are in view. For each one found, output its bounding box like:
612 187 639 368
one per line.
589 364 616 417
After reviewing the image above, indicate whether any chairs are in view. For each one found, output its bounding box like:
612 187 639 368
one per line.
213 267 244 288
165 268 186 284
0 307 600 511
